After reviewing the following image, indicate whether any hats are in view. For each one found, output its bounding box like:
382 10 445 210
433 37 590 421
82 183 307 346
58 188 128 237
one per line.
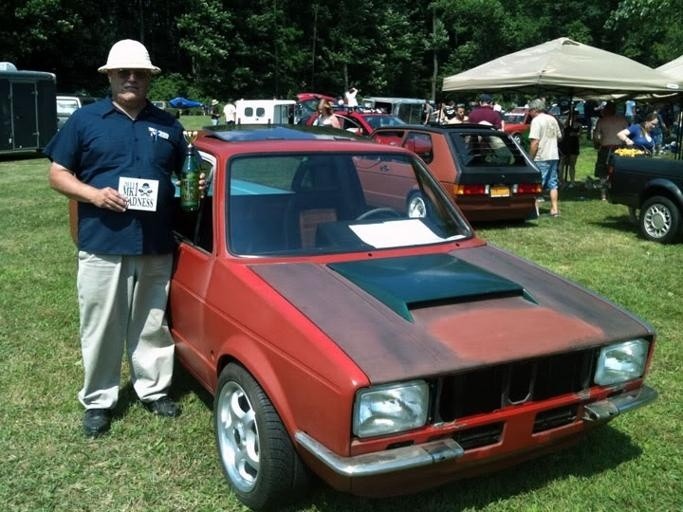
97 39 161 75
528 99 541 112
211 99 218 106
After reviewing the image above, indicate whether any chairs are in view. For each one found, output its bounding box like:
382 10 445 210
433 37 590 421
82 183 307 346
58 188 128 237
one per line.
283 160 352 249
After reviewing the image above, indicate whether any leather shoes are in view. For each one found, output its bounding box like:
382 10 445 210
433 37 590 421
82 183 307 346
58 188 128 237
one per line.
83 409 110 435
141 396 179 417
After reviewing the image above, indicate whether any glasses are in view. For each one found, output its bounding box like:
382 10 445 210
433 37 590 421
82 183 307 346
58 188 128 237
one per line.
118 70 147 79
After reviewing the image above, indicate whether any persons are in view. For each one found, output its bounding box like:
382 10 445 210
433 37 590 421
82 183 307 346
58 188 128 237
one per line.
309 98 339 129
617 113 663 226
415 96 683 185
345 87 359 106
210 98 221 125
223 97 236 125
529 101 562 217
42 39 207 435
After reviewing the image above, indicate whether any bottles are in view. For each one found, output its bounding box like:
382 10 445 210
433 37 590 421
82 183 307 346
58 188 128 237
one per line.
180 145 201 213
